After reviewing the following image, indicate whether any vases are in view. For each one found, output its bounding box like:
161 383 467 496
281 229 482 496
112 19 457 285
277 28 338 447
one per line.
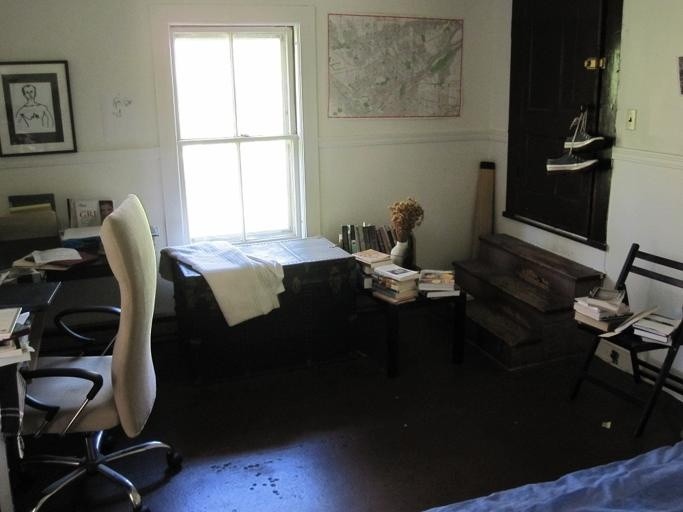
390 239 414 267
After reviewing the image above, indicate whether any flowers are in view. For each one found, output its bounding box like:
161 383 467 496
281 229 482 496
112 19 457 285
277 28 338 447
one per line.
386 194 424 242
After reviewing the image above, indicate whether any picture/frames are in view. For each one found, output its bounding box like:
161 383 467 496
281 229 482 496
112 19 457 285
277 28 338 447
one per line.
0 61 77 157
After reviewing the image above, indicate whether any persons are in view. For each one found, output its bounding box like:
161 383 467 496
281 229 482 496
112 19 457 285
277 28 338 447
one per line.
98 200 113 220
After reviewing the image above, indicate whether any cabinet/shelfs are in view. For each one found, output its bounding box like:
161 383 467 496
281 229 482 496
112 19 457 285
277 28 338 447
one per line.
173 236 357 377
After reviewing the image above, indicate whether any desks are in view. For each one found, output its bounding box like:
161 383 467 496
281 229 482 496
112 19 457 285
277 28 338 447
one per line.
0 234 114 511
354 265 467 363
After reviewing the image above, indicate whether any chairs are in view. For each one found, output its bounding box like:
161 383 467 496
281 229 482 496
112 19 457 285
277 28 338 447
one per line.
567 243 683 436
20 193 183 512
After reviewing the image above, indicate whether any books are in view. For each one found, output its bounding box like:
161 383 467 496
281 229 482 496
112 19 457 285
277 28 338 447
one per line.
66 198 101 228
336 221 461 306
567 285 682 348
0 307 35 367
10 248 83 274
